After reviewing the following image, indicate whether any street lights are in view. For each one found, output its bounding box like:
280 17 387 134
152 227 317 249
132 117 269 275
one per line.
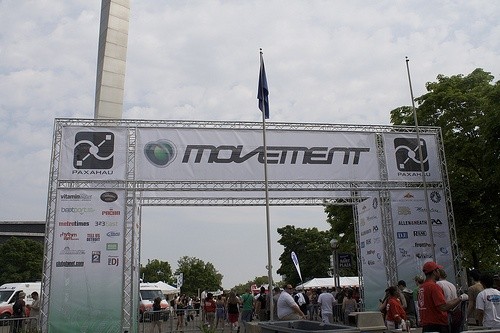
330 238 339 287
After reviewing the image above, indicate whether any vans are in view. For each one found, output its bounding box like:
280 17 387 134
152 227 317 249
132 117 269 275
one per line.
0 283 41 326
139 282 171 322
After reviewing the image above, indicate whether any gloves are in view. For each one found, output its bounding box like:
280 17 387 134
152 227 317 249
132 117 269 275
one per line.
461 293 469 301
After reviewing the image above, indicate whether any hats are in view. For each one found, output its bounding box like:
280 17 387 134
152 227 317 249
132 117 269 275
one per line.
19 292 26 298
412 275 424 282
396 280 406 286
423 262 444 274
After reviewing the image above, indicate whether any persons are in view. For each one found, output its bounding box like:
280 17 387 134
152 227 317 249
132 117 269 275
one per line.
148 297 163 333
9 292 40 333
166 284 364 333
379 275 424 333
465 270 485 326
475 271 500 329
435 269 462 333
417 262 469 333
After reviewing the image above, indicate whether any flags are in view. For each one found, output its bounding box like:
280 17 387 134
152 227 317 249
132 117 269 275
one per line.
257 56 269 119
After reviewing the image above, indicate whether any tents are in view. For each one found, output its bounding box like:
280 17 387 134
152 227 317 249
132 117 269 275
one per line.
157 280 178 301
295 277 363 290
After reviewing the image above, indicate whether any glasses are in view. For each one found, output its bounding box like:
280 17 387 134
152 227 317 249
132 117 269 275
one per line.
287 288 293 290
